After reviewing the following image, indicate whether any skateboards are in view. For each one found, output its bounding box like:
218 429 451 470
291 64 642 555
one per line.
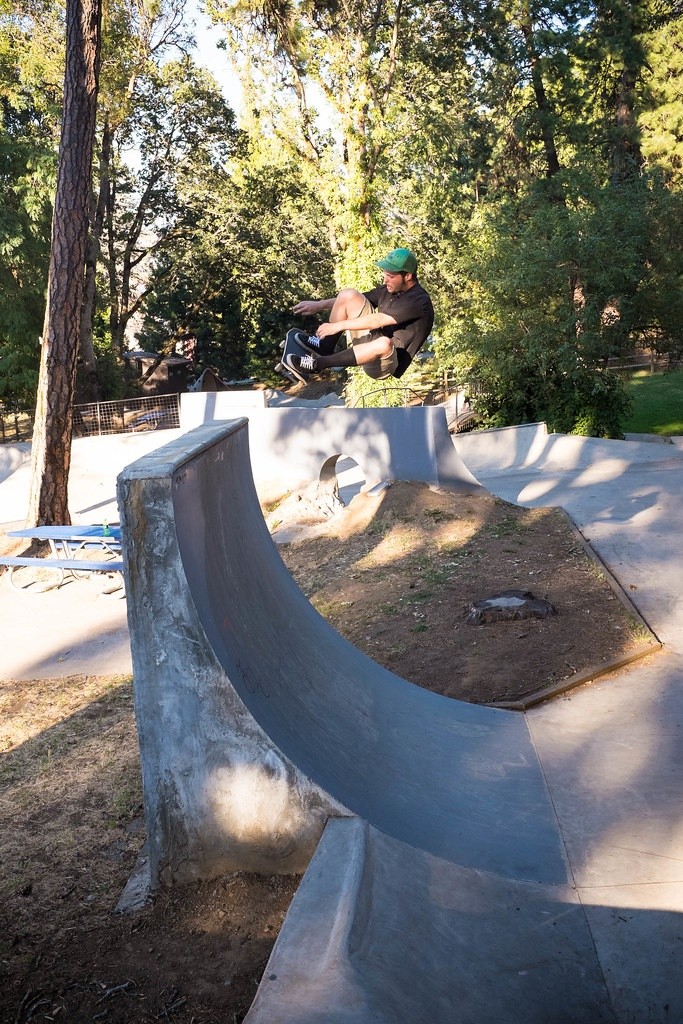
273 325 315 392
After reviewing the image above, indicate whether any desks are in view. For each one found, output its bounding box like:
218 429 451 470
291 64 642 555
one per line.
4 525 126 598
137 412 166 422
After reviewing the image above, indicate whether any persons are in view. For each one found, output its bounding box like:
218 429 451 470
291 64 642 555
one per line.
287 249 434 382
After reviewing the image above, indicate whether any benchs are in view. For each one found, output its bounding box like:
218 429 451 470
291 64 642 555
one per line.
54 541 123 550
0 556 124 572
130 418 163 430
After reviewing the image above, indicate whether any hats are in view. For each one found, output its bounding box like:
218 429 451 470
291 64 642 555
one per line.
377 248 418 274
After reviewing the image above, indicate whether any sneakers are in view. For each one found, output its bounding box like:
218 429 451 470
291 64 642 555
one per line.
287 353 321 375
294 332 332 357
